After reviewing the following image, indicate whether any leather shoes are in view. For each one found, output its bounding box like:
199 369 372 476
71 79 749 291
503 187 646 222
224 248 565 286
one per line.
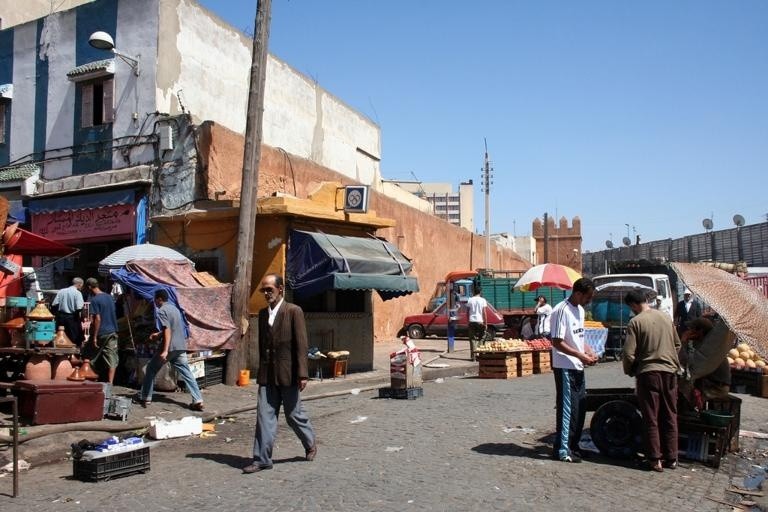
306 440 318 461
243 461 274 473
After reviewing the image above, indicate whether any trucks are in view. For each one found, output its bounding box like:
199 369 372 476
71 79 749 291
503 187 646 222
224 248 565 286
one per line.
590 264 768 362
424 268 592 338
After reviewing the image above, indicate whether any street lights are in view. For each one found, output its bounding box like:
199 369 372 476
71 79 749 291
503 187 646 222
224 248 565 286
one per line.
625 223 631 246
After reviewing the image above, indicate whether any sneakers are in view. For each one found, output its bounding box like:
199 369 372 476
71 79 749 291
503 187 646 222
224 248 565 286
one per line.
553 444 677 472
141 396 153 407
189 400 207 410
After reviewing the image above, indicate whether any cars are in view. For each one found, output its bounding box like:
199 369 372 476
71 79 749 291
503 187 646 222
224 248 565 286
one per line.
403 297 505 341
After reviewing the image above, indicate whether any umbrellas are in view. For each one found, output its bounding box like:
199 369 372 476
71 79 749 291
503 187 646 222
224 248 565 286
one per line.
669 261 768 363
513 263 583 307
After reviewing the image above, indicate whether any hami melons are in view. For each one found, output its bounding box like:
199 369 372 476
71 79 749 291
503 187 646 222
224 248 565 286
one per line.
726 344 765 369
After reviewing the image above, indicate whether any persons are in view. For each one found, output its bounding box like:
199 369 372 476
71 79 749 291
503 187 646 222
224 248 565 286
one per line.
133 289 205 411
533 295 552 338
677 289 702 336
50 277 84 359
622 286 682 472
243 274 317 475
547 277 599 463
465 286 487 362
80 277 119 384
678 317 732 401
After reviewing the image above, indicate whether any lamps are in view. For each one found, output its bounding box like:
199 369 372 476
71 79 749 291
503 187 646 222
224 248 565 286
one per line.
88 31 141 76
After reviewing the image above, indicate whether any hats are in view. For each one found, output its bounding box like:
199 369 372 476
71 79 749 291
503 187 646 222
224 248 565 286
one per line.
533 294 546 303
655 288 692 300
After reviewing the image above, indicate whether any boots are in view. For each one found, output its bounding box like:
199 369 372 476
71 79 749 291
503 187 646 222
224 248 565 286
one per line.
106 367 117 386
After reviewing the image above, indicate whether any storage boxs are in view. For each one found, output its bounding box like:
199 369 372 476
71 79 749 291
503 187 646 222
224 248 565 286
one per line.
14 379 105 425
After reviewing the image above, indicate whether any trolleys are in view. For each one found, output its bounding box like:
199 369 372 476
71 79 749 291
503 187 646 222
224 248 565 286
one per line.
552 386 642 459
603 323 630 362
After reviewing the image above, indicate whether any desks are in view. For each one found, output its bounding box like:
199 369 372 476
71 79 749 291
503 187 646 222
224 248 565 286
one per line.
0 347 80 397
